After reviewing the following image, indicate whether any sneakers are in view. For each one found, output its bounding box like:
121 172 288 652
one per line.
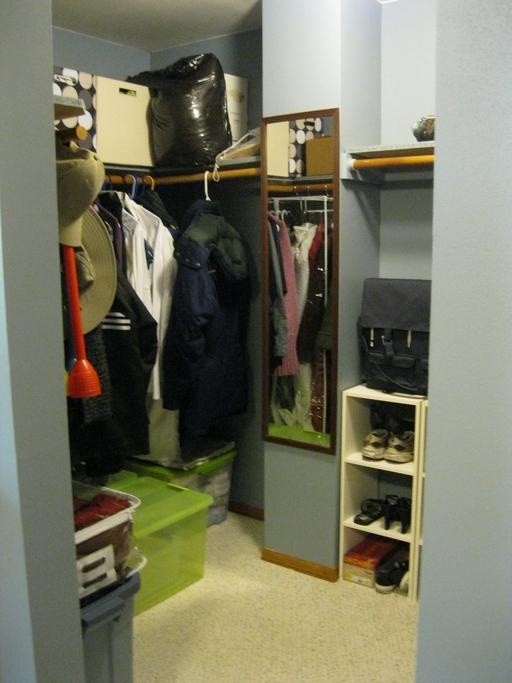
375 559 409 591
361 405 415 463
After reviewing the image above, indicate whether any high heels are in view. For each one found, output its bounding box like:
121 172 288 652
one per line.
354 495 411 534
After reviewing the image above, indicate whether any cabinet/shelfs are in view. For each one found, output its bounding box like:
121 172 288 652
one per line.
338 382 429 602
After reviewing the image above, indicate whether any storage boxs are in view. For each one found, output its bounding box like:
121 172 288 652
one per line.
104 469 213 617
73 479 142 599
117 442 238 527
77 573 142 683
53 66 246 169
266 117 334 177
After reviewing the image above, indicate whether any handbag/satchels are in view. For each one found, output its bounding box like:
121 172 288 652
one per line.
356 277 432 396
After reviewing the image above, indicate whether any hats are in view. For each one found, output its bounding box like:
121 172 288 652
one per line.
56 141 117 341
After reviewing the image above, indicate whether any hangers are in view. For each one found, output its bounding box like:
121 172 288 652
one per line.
268 199 310 221
103 169 216 201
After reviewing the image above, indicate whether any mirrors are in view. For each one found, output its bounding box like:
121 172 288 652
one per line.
262 109 340 457
